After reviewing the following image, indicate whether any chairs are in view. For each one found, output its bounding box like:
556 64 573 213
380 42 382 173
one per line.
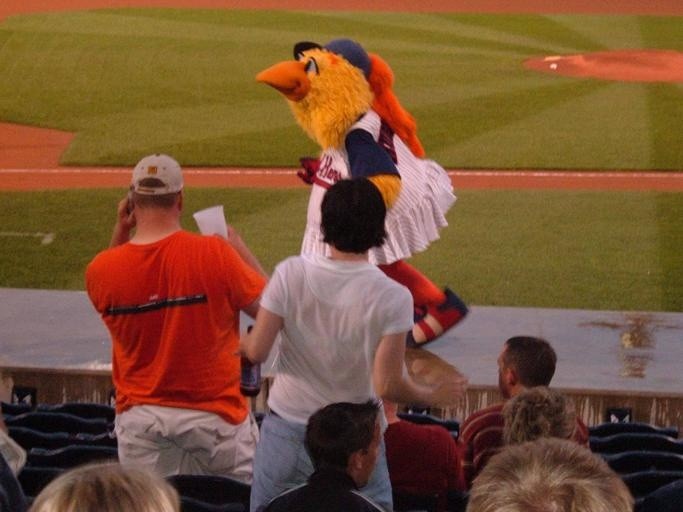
0 402 682 512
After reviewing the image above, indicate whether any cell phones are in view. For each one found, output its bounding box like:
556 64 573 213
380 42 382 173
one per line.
129 188 133 211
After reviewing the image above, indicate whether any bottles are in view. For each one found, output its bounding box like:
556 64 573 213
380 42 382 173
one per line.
240 325 260 395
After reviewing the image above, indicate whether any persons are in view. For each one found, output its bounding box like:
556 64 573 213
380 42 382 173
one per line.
84 154 268 487
385 337 634 511
257 396 385 512
31 462 180 511
236 178 470 512
1 420 30 511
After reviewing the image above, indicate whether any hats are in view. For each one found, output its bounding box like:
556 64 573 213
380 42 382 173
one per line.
128 153 182 197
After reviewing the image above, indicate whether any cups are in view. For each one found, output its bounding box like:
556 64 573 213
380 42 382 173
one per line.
191 204 227 236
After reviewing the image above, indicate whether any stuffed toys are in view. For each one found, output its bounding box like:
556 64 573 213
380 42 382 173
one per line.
253 36 468 350
